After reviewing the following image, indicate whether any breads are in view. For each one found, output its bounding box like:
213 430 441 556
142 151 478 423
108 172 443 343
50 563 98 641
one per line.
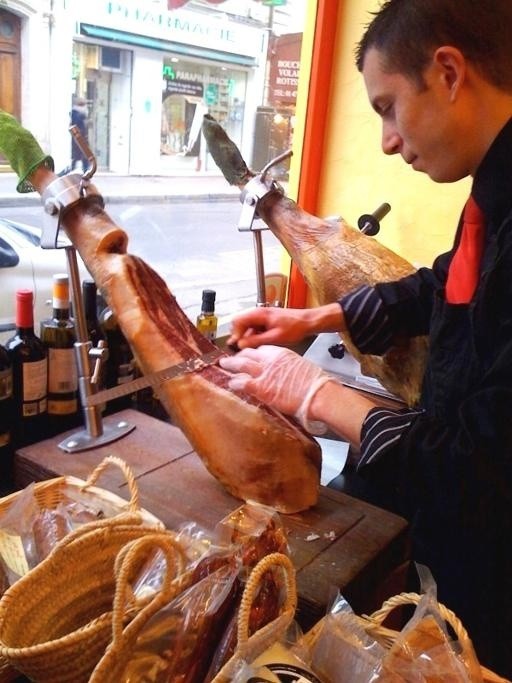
384 613 465 683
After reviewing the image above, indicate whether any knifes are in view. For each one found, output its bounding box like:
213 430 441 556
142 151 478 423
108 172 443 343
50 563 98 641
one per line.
80 335 240 415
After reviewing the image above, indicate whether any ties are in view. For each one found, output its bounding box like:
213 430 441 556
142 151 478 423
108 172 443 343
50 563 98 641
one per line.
444 194 486 305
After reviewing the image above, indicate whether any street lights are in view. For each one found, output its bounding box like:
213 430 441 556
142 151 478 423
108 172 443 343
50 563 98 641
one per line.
274 114 295 150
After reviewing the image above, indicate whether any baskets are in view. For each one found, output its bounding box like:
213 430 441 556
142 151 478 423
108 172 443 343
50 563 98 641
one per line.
0 454 480 681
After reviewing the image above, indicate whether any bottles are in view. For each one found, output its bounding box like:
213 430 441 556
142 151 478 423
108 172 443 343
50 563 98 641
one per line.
196 290 217 339
0 274 115 432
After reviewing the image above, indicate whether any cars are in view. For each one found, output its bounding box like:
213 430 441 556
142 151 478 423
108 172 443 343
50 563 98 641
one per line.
0 218 100 368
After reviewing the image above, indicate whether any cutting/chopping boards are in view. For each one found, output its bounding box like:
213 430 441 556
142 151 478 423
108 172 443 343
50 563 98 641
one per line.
16 409 407 612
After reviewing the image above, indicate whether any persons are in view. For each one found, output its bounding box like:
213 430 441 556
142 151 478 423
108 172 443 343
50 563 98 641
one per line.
219 0 512 676
72 95 90 174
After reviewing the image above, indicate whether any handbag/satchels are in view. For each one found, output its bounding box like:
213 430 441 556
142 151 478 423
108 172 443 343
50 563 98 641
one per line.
0 454 512 683
